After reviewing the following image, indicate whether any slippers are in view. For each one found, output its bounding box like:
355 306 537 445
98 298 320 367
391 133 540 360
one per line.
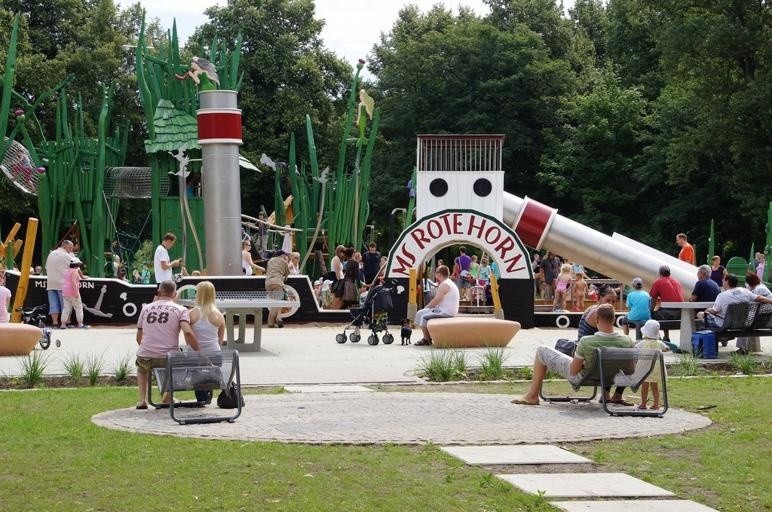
510 396 541 406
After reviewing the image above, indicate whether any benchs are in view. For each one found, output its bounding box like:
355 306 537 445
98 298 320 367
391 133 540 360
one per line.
704 302 772 339
0 322 42 355
426 317 521 348
630 315 705 343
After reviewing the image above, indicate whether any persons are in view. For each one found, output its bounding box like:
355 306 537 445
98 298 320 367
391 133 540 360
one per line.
632 318 669 410
510 303 635 406
183 281 227 408
0 230 201 331
575 287 635 407
131 279 214 409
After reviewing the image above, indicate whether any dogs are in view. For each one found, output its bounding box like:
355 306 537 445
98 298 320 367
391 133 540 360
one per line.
400 318 413 347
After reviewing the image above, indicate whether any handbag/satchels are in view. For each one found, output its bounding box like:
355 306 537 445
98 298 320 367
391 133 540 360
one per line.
459 269 476 284
330 278 345 298
217 380 246 409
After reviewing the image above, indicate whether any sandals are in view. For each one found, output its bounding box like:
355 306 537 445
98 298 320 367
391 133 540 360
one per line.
414 337 431 346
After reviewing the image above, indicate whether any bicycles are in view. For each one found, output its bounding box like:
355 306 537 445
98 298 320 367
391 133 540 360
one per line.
15 303 61 351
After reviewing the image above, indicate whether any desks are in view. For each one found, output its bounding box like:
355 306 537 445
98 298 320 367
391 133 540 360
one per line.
662 299 719 352
177 290 300 352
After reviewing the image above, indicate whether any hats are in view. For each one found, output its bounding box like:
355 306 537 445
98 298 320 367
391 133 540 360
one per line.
275 249 291 257
630 277 644 289
658 264 671 276
640 319 661 340
69 256 84 264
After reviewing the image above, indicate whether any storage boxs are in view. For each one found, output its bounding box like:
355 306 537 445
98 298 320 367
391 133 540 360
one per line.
689 329 719 359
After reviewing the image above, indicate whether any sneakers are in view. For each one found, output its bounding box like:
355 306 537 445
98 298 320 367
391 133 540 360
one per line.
52 322 93 330
267 318 284 329
135 402 150 410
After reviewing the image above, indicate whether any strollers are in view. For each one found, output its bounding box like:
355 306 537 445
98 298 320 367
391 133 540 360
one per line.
333 279 401 346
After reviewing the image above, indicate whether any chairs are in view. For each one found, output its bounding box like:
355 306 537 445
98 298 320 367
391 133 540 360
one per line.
145 349 246 425
541 347 670 417
